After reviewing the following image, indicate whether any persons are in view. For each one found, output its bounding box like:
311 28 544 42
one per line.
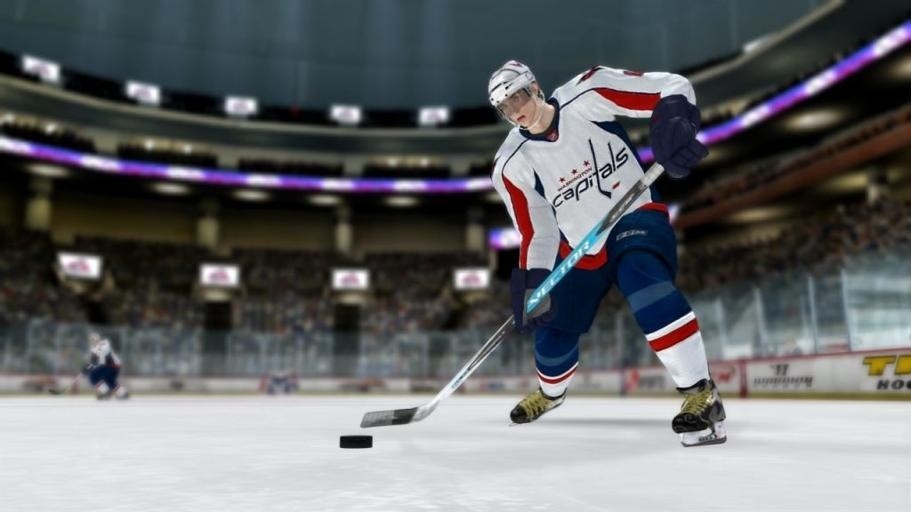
2 194 909 377
487 59 726 432
82 331 130 401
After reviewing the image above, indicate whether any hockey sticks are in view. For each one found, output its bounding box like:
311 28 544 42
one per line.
359 162 666 430
